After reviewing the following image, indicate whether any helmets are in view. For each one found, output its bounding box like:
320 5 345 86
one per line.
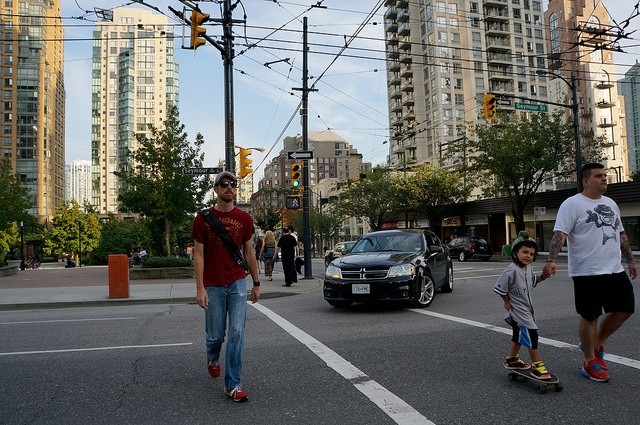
510 230 538 252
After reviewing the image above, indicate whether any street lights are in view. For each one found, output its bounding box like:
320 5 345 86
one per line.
20 220 25 270
536 70 584 194
235 147 266 160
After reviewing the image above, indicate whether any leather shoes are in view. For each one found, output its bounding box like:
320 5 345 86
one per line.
268 277 272 280
282 283 291 286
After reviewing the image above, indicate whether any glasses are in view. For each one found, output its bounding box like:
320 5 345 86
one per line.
218 179 237 188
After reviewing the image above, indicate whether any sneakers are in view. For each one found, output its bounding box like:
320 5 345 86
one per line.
579 341 609 369
502 353 532 370
206 355 221 378
580 358 611 381
224 385 249 402
529 359 552 379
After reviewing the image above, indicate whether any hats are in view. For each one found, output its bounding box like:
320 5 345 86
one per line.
215 170 237 184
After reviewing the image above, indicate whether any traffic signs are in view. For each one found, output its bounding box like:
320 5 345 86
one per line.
514 102 548 112
183 167 223 174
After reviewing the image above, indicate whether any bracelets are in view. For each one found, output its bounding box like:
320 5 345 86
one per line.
540 274 545 281
624 257 637 264
546 259 556 263
253 280 260 287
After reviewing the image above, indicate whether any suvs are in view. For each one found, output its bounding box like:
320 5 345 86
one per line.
447 236 492 262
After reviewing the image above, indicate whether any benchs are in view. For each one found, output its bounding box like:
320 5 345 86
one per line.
26 261 41 270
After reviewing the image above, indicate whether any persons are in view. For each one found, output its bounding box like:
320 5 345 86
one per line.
492 230 553 379
255 238 263 274
189 170 262 403
287 225 298 269
259 230 277 281
66 256 75 268
129 247 141 266
323 245 329 255
139 247 147 258
543 162 638 383
32 254 41 269
26 254 34 266
272 227 300 288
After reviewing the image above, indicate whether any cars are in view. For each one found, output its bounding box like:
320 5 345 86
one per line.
325 241 357 271
323 228 453 309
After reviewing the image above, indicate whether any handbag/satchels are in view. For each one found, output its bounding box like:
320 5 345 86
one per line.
199 207 250 273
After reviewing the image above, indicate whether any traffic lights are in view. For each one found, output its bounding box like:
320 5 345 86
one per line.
483 94 497 120
240 148 253 178
290 163 301 189
286 196 300 209
190 11 210 49
